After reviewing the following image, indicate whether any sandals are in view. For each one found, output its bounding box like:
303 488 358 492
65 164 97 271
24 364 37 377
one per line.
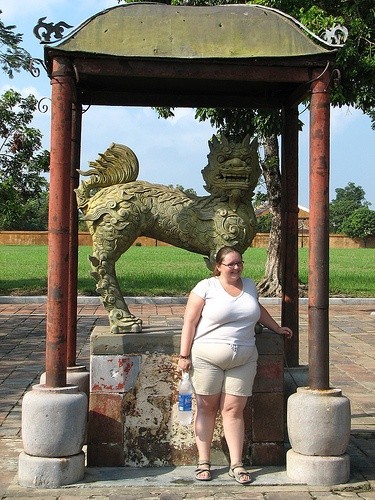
229 462 252 484
195 461 212 480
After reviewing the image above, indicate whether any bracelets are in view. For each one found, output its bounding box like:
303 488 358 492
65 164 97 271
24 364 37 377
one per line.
178 353 190 358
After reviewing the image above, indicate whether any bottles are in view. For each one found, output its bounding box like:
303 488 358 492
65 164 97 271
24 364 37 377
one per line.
178 372 192 427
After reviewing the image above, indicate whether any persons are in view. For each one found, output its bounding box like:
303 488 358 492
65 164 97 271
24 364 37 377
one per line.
176 246 293 483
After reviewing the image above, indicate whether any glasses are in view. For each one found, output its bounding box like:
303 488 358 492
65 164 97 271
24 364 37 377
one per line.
221 260 244 268
